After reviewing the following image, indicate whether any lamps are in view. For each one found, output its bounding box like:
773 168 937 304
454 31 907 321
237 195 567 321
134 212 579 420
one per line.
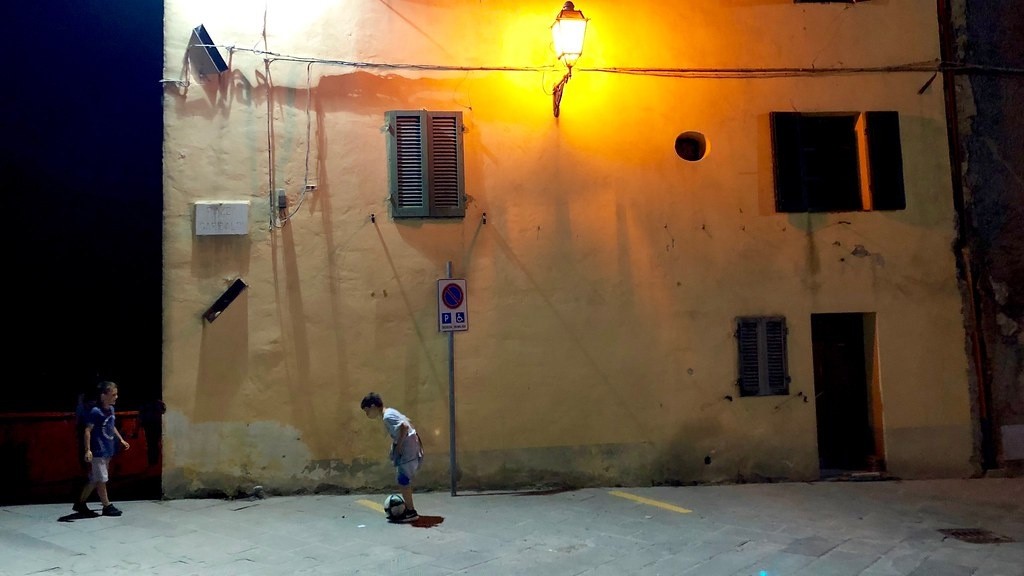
550 0 591 118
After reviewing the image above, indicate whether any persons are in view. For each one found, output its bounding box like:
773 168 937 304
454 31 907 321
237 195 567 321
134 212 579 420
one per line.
71 380 131 516
360 391 425 524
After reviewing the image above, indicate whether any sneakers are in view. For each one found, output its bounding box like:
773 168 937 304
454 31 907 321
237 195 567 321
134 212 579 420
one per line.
389 509 419 522
73 502 94 515
103 504 122 516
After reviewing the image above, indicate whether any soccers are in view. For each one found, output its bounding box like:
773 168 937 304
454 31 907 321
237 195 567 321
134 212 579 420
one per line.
383 494 406 518
